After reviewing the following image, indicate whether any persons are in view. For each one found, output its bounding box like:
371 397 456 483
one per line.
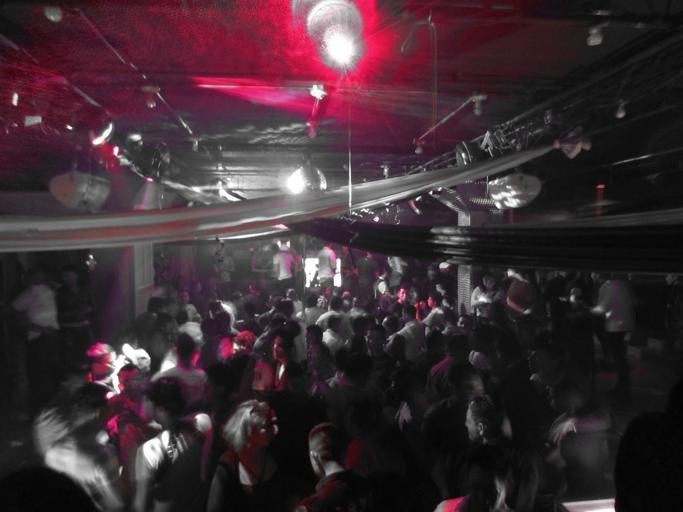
1 236 683 512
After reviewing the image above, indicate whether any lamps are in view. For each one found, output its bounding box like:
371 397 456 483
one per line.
43 0 202 152
400 7 487 155
487 147 542 210
47 145 112 213
